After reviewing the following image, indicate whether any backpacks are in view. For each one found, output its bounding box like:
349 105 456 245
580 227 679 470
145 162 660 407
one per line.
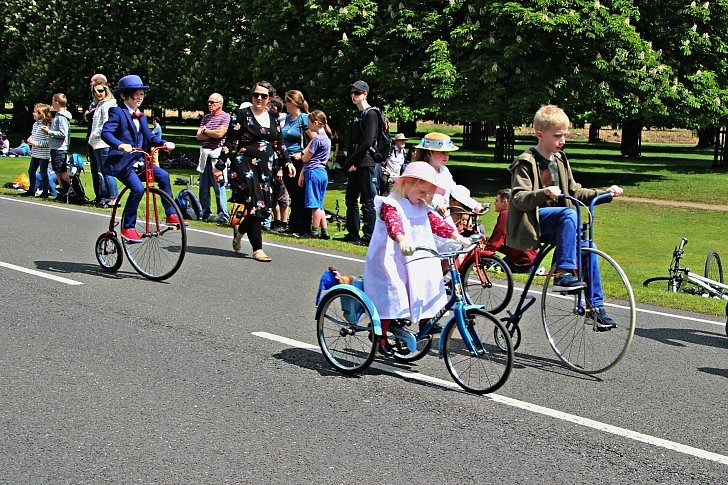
359 107 391 164
68 152 84 170
175 188 203 221
54 174 90 205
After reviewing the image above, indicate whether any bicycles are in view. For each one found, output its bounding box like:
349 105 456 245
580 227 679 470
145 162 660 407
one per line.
159 151 199 169
95 146 188 282
322 199 365 232
643 238 728 301
314 191 636 395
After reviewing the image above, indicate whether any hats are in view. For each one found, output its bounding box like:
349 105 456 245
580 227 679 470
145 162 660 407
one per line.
348 80 370 93
116 74 149 91
394 133 409 140
389 161 446 196
414 132 459 152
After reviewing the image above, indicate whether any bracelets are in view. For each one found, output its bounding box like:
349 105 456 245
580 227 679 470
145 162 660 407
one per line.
202 129 206 135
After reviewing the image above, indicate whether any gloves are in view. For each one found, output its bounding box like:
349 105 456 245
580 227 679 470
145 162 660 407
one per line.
454 235 473 248
398 238 416 256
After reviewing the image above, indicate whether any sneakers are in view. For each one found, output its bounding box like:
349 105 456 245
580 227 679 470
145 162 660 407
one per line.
584 308 617 328
165 213 190 226
550 272 588 292
121 227 142 243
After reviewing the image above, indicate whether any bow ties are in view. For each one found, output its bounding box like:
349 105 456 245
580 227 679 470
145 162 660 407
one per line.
131 111 145 120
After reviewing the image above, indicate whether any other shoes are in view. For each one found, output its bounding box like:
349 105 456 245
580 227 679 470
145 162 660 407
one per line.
263 222 287 233
20 187 35 197
297 231 330 240
84 198 108 206
35 191 49 198
277 228 299 237
51 193 64 202
353 235 371 246
332 233 360 242
99 200 121 207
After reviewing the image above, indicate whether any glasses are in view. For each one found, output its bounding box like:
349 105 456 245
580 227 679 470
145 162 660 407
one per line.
94 90 104 93
207 101 219 105
251 92 269 99
350 91 364 96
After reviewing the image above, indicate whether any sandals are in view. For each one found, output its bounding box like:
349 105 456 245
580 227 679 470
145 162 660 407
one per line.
252 249 273 262
232 232 241 252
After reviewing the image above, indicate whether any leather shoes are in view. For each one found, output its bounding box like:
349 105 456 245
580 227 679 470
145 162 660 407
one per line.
418 320 443 335
368 331 394 357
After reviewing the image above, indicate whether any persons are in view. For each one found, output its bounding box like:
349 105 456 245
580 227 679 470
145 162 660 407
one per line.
100 75 189 243
196 93 231 223
506 104 623 328
150 117 162 167
85 74 121 208
41 93 72 202
364 161 473 357
370 132 416 197
271 90 344 240
212 81 296 262
337 80 379 247
21 103 53 198
449 185 539 274
414 132 484 290
35 153 73 196
0 131 30 157
